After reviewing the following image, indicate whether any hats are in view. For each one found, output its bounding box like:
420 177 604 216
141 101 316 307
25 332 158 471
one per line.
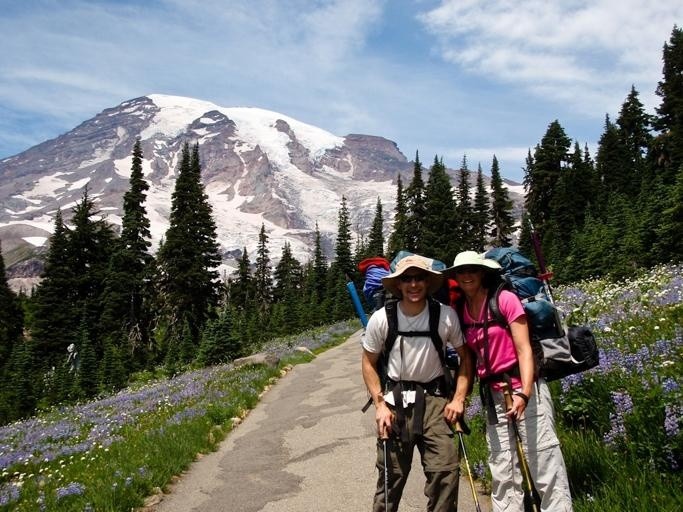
381 251 501 298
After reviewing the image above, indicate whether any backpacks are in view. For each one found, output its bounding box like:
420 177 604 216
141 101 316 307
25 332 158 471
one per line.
356 256 445 365
454 246 599 383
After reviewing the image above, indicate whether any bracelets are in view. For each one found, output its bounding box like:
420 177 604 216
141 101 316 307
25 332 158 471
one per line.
515 392 529 408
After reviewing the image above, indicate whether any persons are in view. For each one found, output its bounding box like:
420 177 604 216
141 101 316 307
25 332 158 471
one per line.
360 250 475 512
440 249 574 512
